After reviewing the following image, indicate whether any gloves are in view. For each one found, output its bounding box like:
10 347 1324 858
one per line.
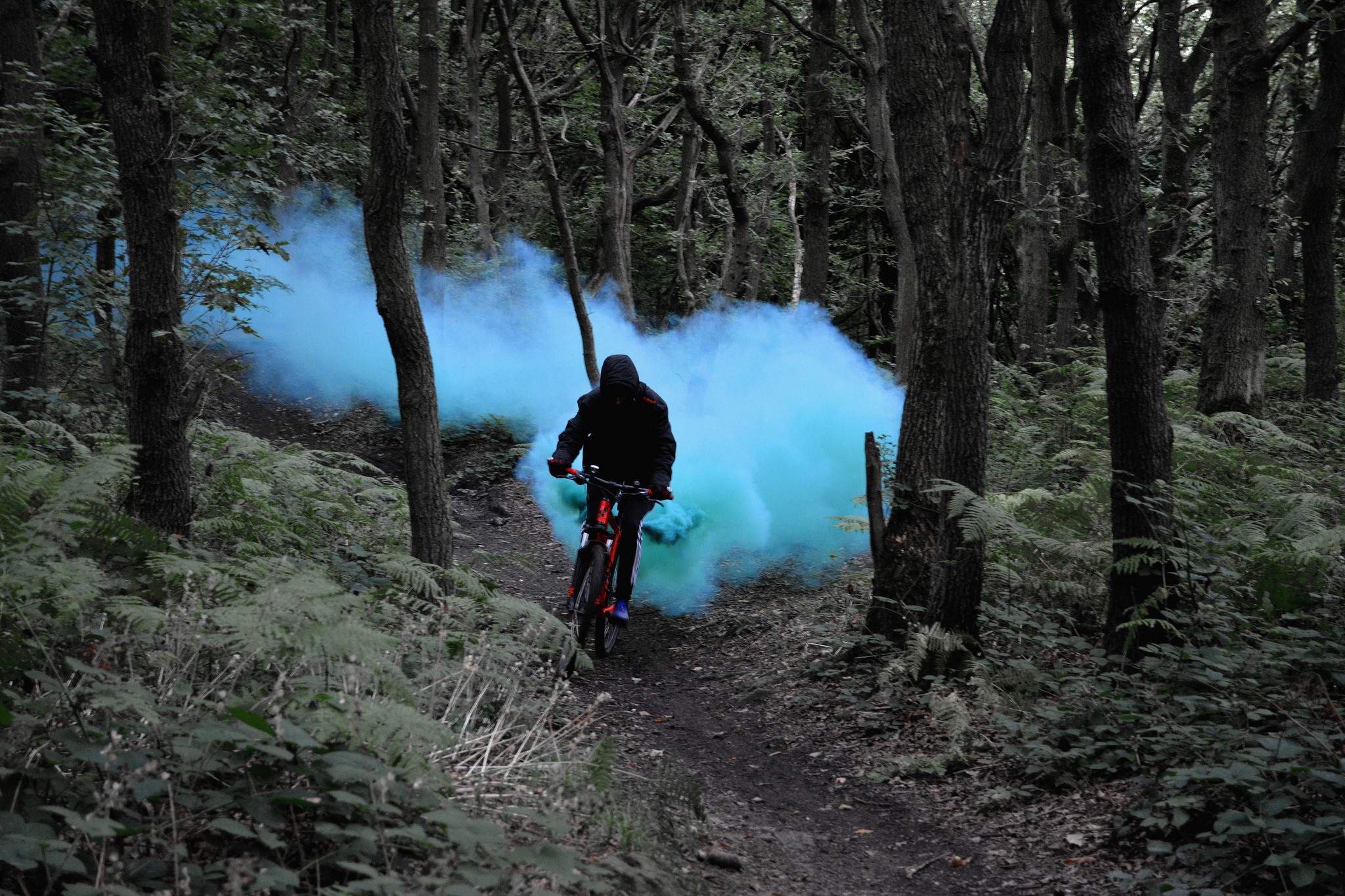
547 457 571 479
649 484 672 501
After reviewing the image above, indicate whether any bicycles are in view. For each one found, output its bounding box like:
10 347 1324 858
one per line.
555 464 674 678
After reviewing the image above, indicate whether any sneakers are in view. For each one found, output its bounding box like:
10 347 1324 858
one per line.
613 600 630 621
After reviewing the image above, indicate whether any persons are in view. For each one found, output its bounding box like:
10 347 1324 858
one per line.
548 355 677 626
